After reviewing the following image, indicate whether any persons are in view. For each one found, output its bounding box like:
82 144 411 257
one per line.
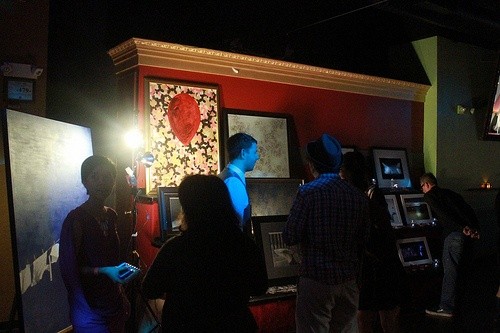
218 132 260 233
59 154 140 333
419 172 480 318
338 152 401 333
282 132 371 333
142 176 270 333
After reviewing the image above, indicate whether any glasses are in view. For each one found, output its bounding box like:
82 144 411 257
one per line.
420 183 425 190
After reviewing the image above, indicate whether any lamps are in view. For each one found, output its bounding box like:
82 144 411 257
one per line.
1 62 44 80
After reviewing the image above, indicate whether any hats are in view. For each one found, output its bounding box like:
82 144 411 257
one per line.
305 134 343 169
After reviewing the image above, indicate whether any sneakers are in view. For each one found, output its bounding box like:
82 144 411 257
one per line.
426 309 453 316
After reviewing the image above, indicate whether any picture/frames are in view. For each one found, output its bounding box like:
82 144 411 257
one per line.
395 236 433 267
251 215 298 288
384 193 403 230
368 146 413 190
222 107 296 179
398 193 434 224
158 186 184 241
143 74 224 201
